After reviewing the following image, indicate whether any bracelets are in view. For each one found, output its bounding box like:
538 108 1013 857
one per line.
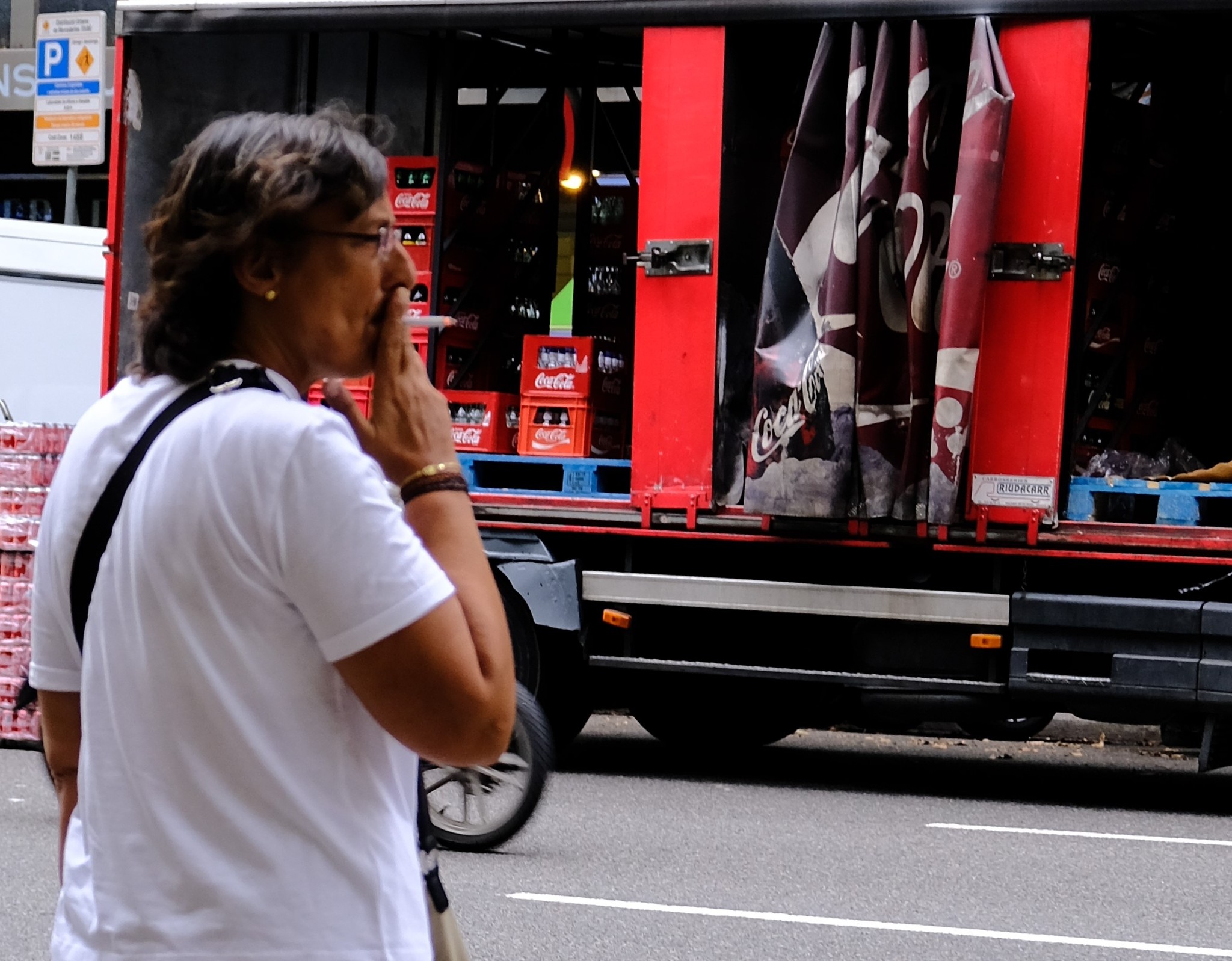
399 462 470 505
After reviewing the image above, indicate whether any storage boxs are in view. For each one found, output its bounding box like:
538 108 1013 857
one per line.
307 156 618 459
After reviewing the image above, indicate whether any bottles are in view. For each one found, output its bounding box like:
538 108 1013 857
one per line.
506 406 519 428
593 415 622 459
447 346 478 369
514 296 542 319
508 238 537 263
403 226 426 246
589 265 621 318
396 167 431 188
592 196 624 250
449 404 484 421
538 346 576 369
594 336 625 394
536 407 569 424
519 182 543 204
457 172 485 195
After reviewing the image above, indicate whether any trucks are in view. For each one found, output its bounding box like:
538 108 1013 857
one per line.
102 0 1232 773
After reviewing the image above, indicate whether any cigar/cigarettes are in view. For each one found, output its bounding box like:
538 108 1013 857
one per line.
400 314 456 326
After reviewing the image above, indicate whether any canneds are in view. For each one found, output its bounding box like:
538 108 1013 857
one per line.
0 421 75 740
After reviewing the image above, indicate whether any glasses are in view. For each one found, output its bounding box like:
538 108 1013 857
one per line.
331 226 402 262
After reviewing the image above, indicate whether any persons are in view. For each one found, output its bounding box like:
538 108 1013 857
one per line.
26 105 515 961
